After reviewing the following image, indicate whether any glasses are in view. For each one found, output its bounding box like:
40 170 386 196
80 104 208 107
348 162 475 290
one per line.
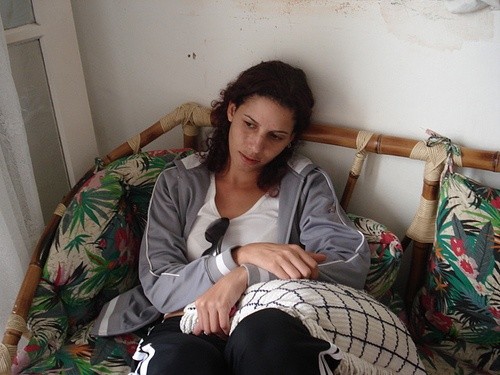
201 217 230 257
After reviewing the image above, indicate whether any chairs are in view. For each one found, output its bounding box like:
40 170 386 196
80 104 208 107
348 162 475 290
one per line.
0 101 500 375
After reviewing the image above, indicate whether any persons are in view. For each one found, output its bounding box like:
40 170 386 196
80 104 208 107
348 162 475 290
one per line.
133 59 370 375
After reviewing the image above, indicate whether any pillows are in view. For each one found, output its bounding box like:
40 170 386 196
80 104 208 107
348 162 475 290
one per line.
347 213 405 300
227 278 427 375
411 172 500 375
